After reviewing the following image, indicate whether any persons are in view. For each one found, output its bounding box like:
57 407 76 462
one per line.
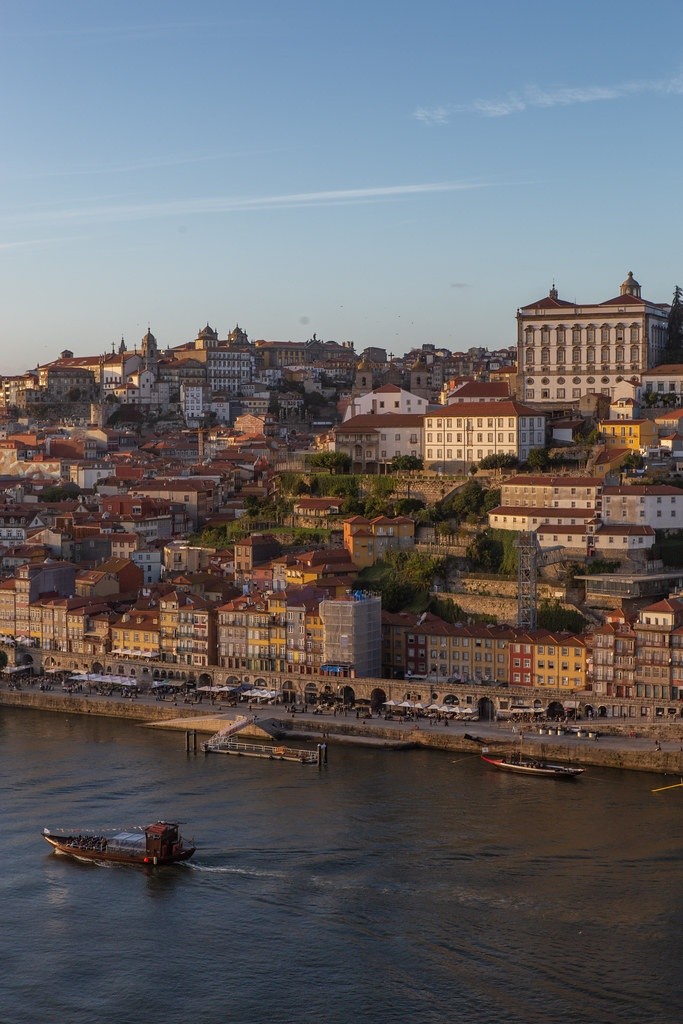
8 676 209 704
226 698 451 730
66 835 108 849
503 711 627 727
500 749 522 763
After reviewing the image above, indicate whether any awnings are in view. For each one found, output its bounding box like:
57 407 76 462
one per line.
69 673 547 715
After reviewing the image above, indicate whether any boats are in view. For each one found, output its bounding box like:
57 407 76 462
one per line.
41 821 197 865
479 731 587 777
